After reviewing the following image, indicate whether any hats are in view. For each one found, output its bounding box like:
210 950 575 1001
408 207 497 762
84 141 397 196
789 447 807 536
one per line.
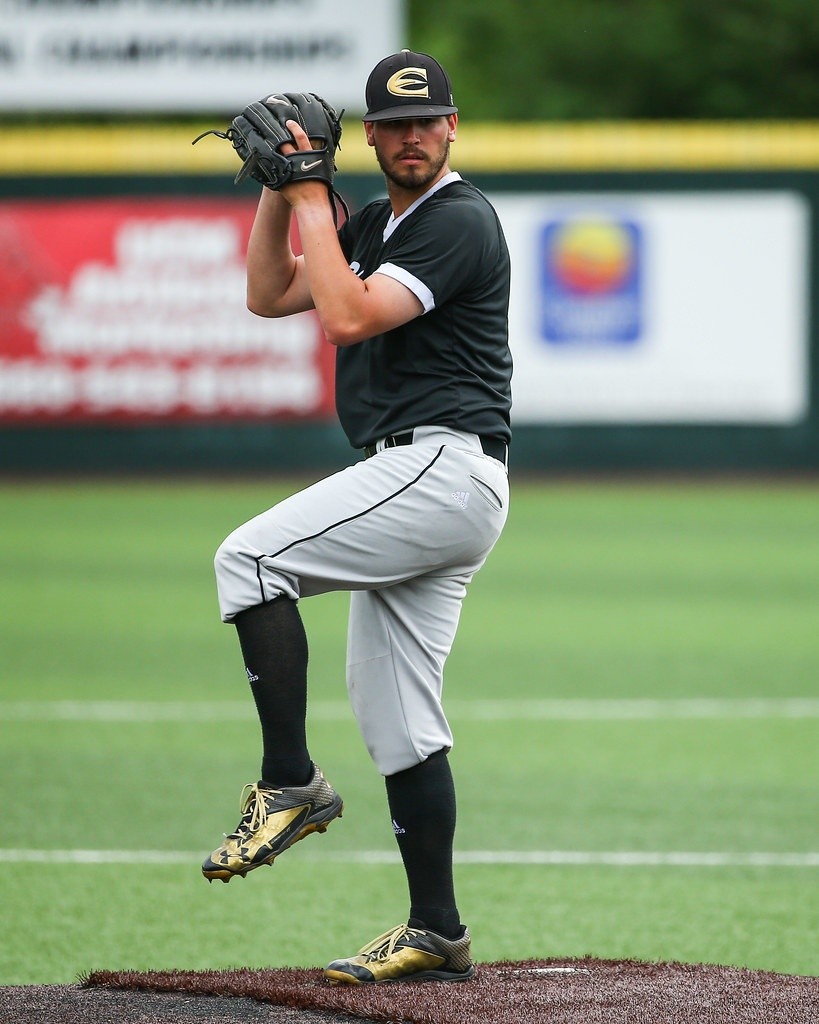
362 52 458 121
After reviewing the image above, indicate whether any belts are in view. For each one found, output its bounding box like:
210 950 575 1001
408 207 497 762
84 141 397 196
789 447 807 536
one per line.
364 431 506 464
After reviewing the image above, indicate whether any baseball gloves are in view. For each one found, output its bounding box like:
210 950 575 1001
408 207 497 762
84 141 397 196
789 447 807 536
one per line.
190 91 353 230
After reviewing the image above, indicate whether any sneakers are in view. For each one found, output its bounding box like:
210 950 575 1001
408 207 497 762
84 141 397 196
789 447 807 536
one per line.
325 926 474 984
202 760 343 883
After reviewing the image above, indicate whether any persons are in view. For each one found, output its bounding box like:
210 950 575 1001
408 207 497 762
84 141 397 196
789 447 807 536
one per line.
202 48 513 982
